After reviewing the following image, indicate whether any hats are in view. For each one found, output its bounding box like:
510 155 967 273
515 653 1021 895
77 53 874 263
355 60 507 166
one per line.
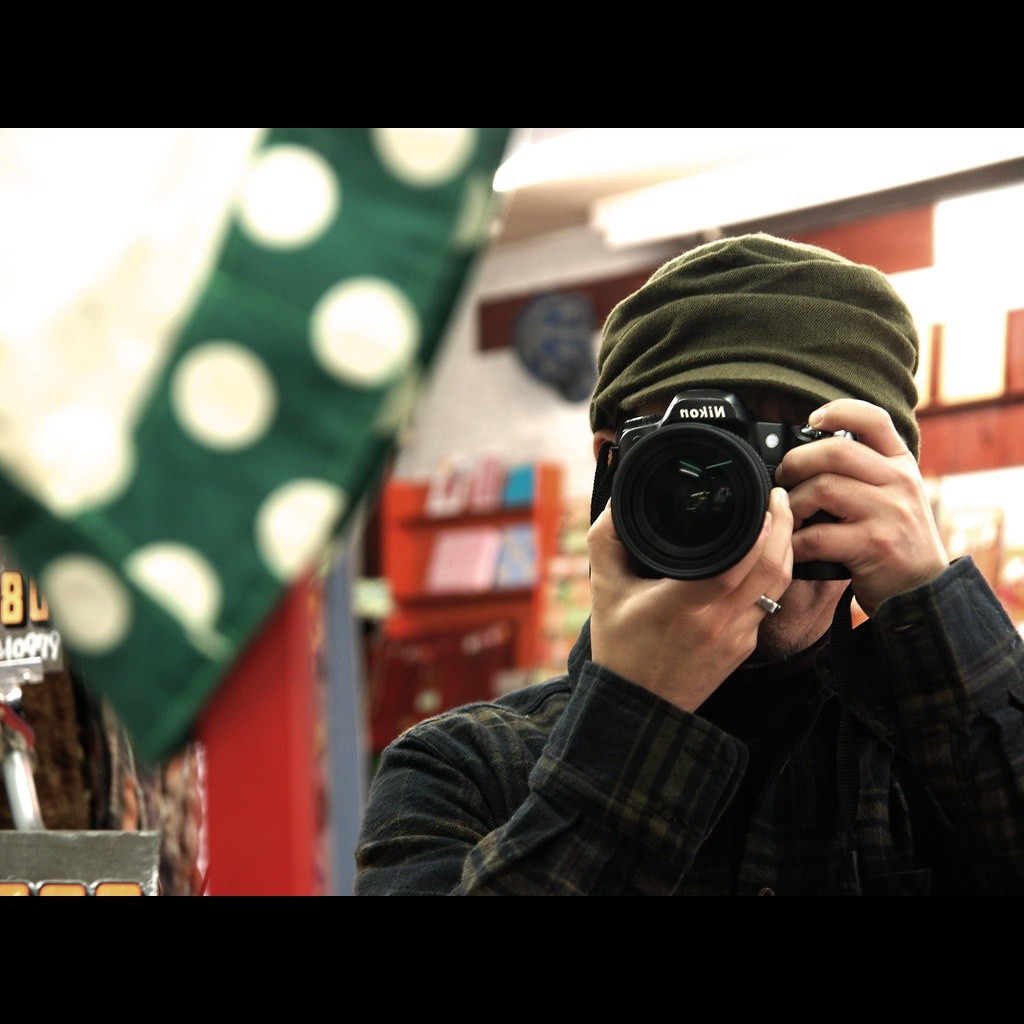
589 231 922 468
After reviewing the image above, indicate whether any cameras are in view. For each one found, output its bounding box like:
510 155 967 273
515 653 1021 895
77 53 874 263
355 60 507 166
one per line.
611 386 855 580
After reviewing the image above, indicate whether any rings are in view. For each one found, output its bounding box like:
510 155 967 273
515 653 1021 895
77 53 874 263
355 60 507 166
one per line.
758 595 781 614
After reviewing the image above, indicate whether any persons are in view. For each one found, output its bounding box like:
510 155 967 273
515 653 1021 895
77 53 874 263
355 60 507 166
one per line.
357 230 1024 897
